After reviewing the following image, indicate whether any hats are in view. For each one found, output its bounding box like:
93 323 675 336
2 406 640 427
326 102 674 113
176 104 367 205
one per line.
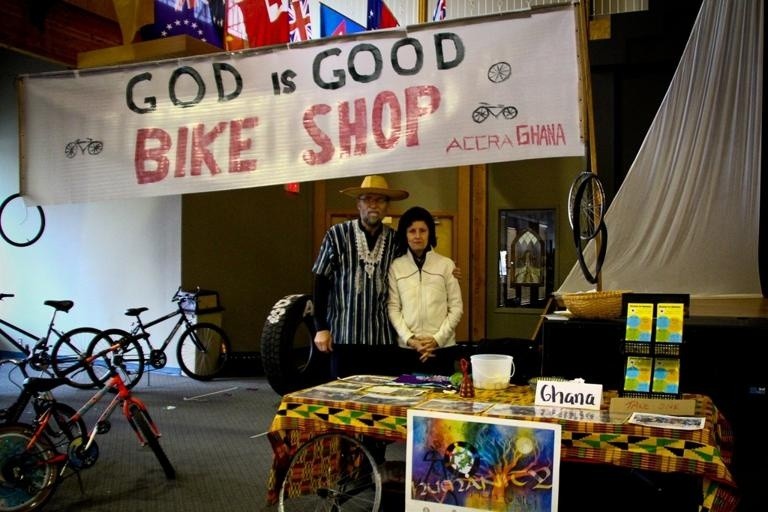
338 176 410 201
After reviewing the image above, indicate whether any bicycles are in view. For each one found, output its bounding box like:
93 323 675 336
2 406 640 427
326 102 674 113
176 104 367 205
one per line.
0 345 176 511
87 288 232 392
0 294 116 389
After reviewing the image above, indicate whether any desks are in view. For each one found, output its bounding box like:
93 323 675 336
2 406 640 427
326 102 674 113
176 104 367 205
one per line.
274 373 723 509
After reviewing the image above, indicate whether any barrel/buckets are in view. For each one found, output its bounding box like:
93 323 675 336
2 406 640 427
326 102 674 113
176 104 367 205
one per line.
470 354 516 391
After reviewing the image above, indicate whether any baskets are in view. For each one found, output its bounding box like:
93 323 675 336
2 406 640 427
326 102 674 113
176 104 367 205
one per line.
561 290 635 319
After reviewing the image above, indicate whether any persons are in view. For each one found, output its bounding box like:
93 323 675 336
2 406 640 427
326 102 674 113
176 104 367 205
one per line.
311 175 462 353
387 206 464 364
